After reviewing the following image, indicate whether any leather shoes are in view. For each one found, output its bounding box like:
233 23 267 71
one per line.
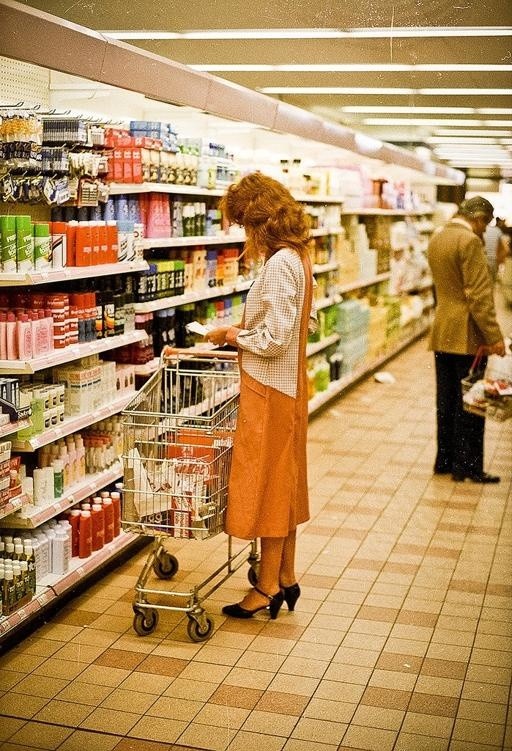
433 460 500 484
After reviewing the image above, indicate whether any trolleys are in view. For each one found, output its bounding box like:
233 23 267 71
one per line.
119 345 261 641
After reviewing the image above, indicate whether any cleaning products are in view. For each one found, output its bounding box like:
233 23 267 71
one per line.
19 482 121 574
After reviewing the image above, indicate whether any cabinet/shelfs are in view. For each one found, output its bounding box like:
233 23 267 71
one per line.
1 102 434 637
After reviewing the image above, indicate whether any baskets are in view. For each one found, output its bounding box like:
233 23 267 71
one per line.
459 342 512 424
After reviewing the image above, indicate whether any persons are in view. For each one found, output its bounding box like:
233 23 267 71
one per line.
203 173 316 622
428 207 511 311
426 196 507 485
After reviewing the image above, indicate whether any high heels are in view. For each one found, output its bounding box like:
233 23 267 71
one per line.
278 581 300 613
222 586 284 620
